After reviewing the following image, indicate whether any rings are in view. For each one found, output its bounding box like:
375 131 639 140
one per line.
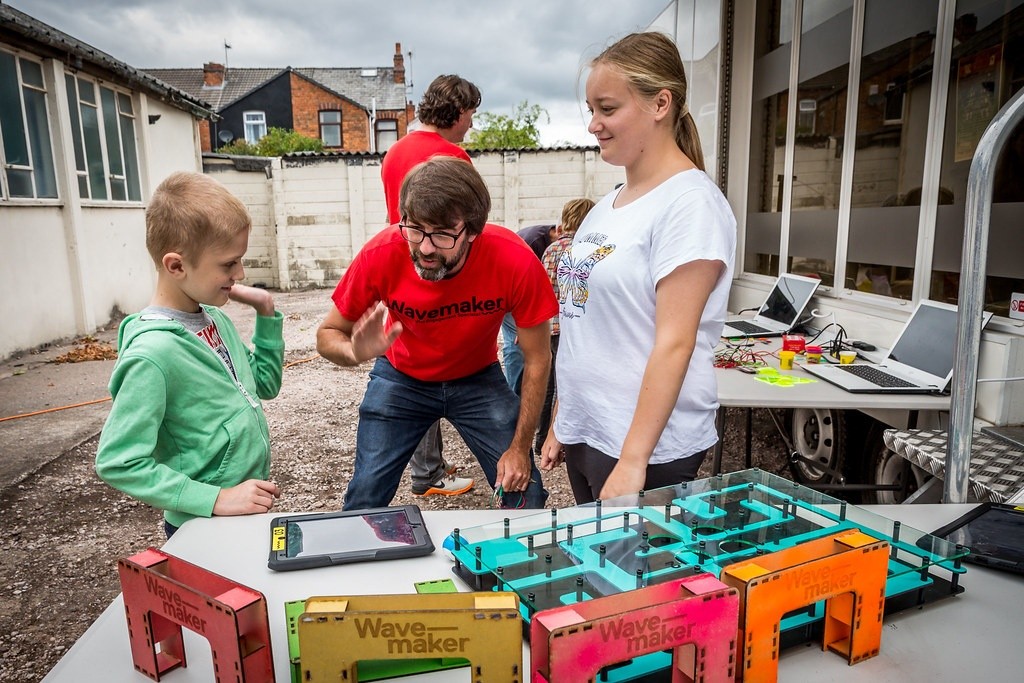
516 486 520 490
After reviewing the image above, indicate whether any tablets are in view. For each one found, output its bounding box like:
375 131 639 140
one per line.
916 502 1024 579
268 504 436 572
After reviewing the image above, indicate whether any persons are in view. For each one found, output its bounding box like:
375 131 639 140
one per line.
315 32 737 512
93 171 286 543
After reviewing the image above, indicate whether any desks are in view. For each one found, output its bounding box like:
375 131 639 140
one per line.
711 314 978 504
39 503 1024 683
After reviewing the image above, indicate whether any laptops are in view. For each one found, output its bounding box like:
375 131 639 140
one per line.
717 272 822 340
800 299 995 392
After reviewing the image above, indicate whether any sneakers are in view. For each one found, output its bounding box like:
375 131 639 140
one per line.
411 475 474 497
440 461 455 475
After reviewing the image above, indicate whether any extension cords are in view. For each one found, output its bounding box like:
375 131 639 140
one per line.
843 339 889 363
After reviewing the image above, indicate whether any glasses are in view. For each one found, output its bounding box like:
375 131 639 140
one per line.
399 214 467 249
